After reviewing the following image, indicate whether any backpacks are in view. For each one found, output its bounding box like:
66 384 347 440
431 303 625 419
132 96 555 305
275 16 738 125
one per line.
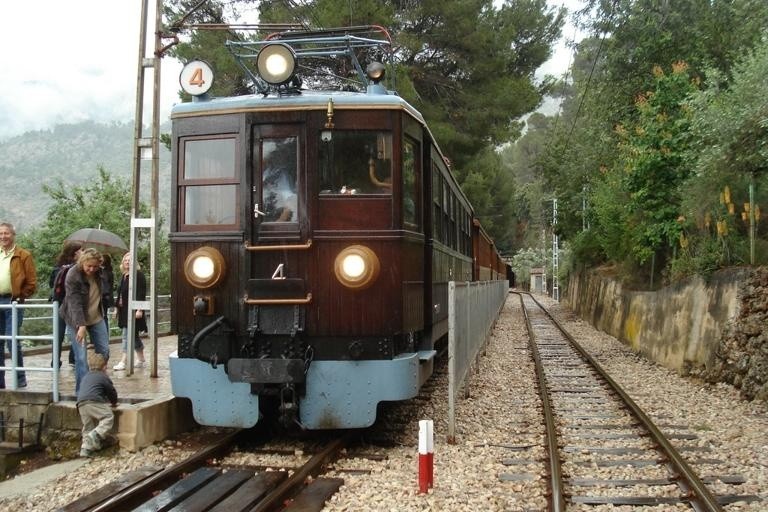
49 263 77 301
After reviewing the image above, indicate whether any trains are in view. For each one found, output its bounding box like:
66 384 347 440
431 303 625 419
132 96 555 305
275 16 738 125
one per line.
148 19 511 441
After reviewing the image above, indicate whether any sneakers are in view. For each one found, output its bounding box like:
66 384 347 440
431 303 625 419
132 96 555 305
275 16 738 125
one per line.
80 430 102 458
113 360 147 370
69 363 75 367
17 373 27 387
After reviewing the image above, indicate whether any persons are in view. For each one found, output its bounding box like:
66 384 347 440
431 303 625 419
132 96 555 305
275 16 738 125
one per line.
0 223 149 457
